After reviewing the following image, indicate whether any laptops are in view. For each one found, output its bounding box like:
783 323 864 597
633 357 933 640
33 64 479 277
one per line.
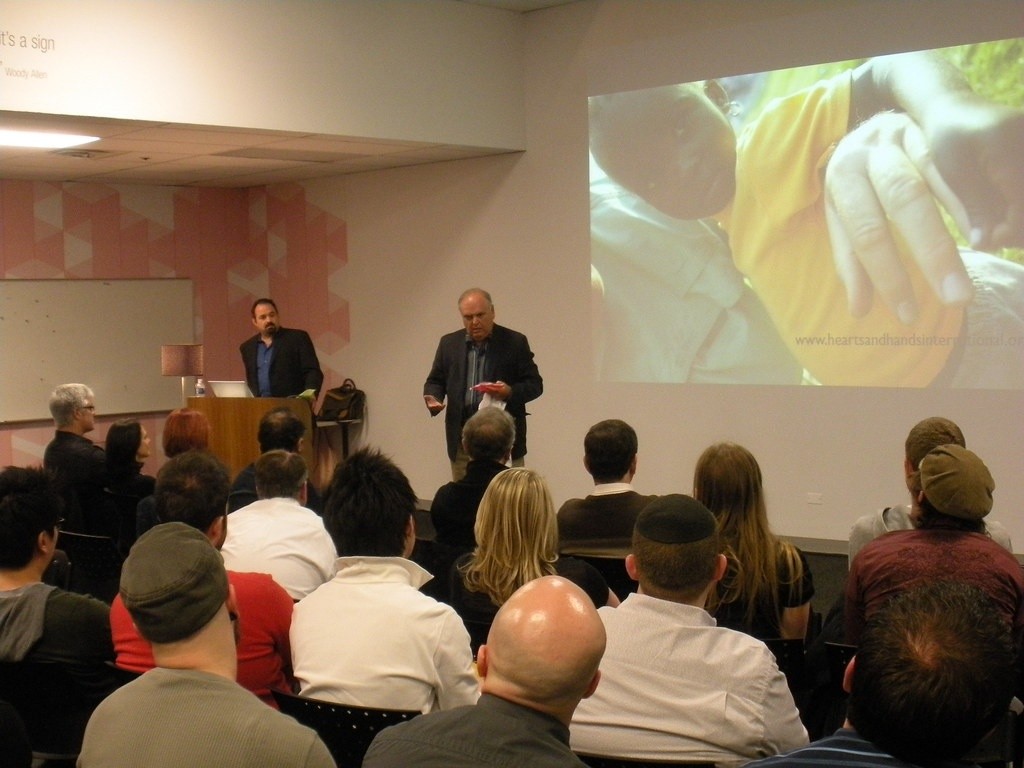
208 381 254 397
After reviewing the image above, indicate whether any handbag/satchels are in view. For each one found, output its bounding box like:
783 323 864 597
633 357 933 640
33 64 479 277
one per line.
320 378 366 419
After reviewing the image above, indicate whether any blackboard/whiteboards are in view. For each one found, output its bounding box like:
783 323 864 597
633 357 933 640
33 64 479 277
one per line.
0 277 194 424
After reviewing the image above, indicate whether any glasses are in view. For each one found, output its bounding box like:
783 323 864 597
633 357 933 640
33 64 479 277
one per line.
80 405 95 415
50 516 65 530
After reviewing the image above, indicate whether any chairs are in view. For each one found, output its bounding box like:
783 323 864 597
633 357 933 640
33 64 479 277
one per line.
1 539 1024 768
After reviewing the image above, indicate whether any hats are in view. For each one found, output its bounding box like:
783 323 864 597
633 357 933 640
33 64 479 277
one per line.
919 442 995 517
636 492 716 545
119 522 230 642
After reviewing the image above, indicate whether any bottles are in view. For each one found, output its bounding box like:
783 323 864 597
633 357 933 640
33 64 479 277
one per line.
195 378 206 396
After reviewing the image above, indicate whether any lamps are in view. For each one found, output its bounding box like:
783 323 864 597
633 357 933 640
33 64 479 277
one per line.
161 343 202 409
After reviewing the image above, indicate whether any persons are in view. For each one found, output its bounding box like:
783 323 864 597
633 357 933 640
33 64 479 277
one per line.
448 467 620 623
556 419 658 556
362 576 607 768
240 298 321 397
0 464 118 754
842 444 1024 650
109 447 294 711
588 50 1024 387
429 407 516 595
569 493 811 768
422 288 543 482
693 442 814 640
218 450 338 599
288 443 480 714
76 522 336 768
740 578 1021 768
43 383 316 514
848 416 1014 572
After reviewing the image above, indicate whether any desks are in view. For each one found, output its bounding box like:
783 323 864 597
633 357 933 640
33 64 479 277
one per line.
316 418 362 461
